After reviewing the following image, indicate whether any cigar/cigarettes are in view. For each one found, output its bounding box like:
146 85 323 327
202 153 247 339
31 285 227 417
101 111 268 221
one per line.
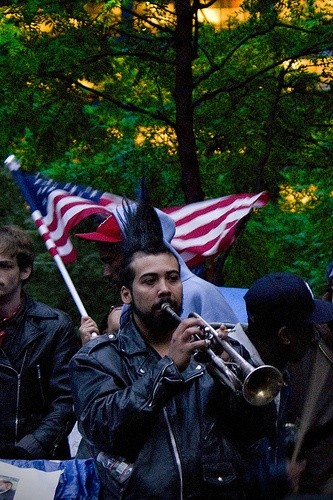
218 329 237 333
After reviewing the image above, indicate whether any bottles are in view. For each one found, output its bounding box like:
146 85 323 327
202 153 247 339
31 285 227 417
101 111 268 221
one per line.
96 451 136 484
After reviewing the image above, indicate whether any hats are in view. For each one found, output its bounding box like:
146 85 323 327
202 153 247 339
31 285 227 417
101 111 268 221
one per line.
243 273 333 323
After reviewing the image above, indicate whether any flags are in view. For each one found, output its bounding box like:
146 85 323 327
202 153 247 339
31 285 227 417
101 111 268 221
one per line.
4 155 270 287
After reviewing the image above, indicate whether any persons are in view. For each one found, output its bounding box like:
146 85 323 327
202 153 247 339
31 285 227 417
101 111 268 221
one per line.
74 203 240 324
0 227 83 460
67 171 333 500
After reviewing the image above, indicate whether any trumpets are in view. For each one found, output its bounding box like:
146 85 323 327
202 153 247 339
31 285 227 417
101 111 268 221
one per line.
161 303 284 407
